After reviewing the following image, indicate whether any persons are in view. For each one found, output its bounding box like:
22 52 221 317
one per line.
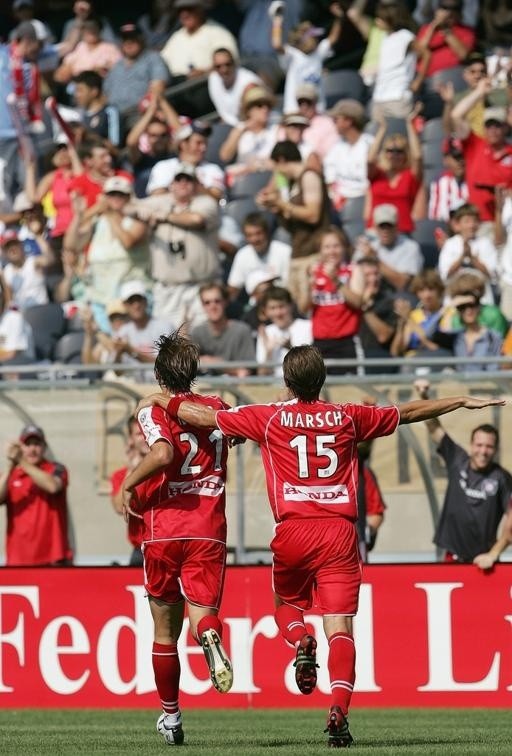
108 413 157 563
118 330 238 745
2 423 70 571
410 368 511 576
130 343 511 749
1 2 512 391
355 437 386 555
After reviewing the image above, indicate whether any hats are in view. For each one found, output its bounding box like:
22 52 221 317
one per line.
105 175 134 196
175 121 213 151
21 423 44 443
1 228 19 248
484 105 508 127
17 20 50 46
170 163 195 182
372 204 397 228
241 87 276 121
105 281 149 319
117 24 143 41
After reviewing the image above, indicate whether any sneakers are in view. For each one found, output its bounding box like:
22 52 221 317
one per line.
324 706 353 748
293 635 320 695
157 713 184 746
202 628 233 693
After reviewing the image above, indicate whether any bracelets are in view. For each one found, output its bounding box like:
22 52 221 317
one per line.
163 394 187 420
